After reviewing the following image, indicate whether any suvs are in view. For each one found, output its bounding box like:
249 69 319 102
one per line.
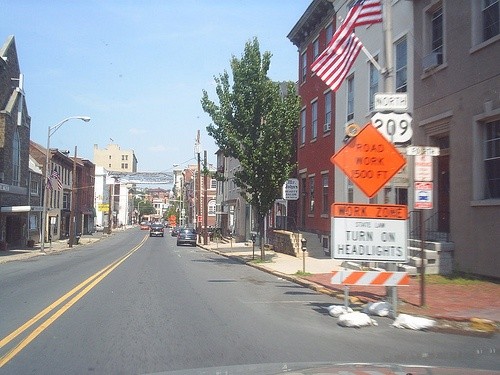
151 223 165 236
176 228 196 247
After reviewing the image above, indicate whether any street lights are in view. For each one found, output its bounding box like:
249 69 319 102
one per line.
138 201 144 211
40 116 90 252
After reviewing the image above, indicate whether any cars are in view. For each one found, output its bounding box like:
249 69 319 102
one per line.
140 220 154 230
171 227 183 236
164 221 172 228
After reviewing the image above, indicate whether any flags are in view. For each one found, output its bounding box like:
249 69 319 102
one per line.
328 0 384 56
309 25 364 93
46 171 63 192
